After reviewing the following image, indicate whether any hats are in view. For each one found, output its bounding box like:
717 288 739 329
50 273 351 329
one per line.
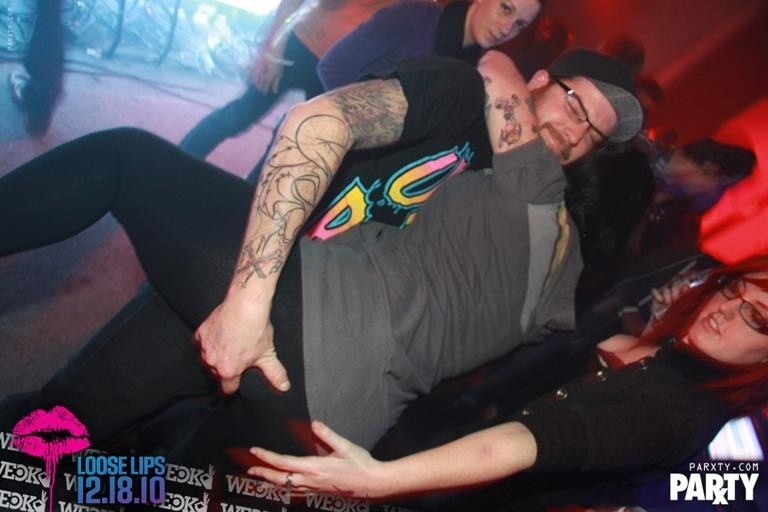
548 48 644 143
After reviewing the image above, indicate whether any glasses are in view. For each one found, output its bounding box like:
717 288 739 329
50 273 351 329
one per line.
719 275 768 335
552 76 605 146
